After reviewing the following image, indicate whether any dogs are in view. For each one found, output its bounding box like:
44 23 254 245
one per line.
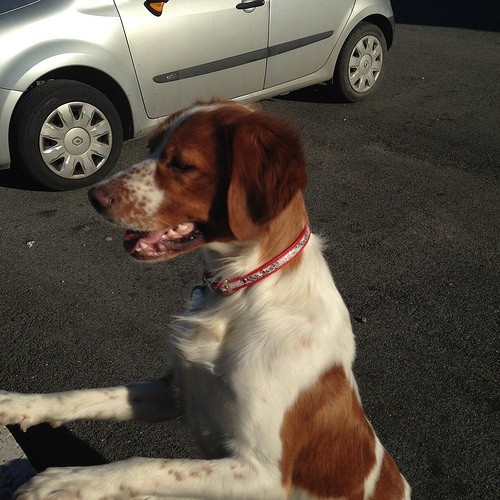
0 97 411 500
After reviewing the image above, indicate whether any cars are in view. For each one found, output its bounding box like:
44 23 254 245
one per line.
1 0 394 191
